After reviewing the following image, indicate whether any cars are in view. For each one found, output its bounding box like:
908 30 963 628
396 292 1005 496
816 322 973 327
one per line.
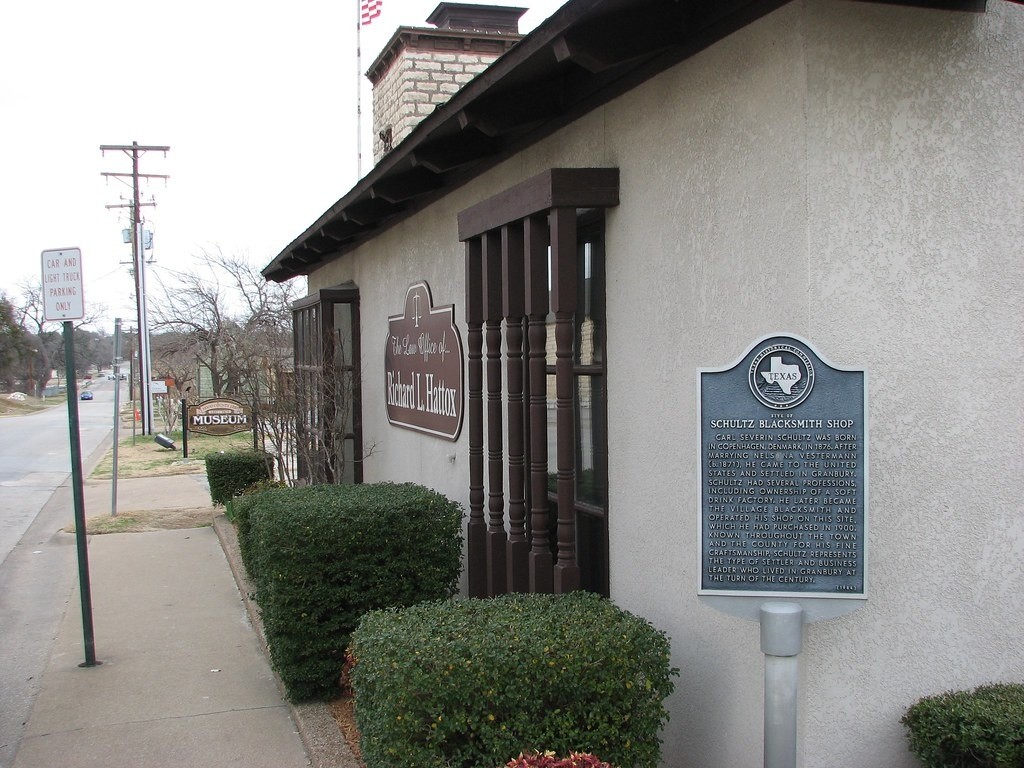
81 390 93 400
108 373 127 381
98 370 105 376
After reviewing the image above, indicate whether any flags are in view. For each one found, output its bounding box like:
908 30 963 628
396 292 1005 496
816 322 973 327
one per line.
361 0 383 25
